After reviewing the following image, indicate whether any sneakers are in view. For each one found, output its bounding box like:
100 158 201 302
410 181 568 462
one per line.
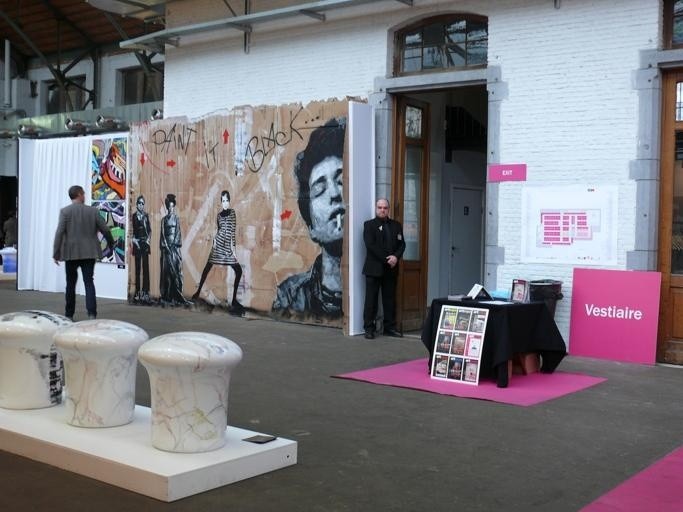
134 292 149 302
192 292 199 297
232 300 243 308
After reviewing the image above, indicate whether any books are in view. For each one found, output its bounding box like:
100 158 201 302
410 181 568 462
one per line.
447 293 472 302
511 278 529 303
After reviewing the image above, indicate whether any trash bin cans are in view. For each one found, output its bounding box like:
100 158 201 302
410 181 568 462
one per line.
528 281 563 325
0 247 17 273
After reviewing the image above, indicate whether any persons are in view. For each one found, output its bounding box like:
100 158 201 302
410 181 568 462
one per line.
52 186 113 322
191 190 243 309
158 194 189 306
130 195 151 304
271 118 345 328
360 198 406 340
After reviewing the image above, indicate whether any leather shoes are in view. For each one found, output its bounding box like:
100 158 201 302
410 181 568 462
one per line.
383 329 403 338
365 330 374 339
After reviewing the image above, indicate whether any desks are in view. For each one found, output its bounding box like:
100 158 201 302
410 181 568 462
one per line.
420 296 566 389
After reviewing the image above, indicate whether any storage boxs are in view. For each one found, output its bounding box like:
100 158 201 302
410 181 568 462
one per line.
512 352 540 375
507 360 512 380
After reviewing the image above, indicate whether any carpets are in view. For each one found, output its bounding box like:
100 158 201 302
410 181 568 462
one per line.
329 356 609 408
